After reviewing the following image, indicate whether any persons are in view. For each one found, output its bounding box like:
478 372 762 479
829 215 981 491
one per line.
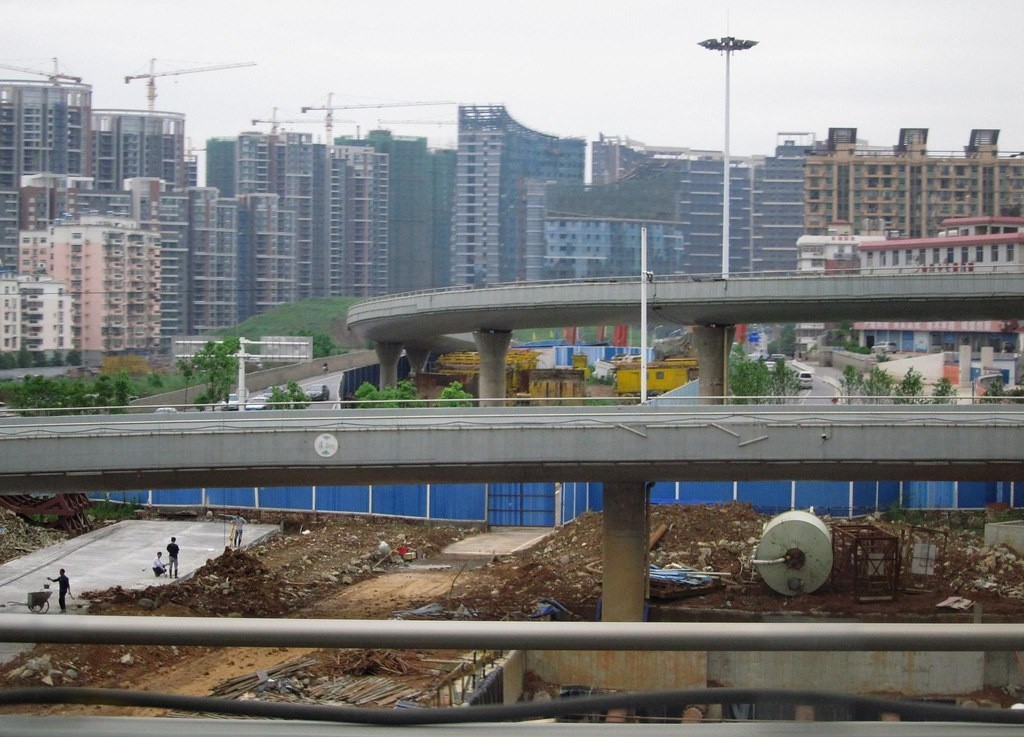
47 569 71 613
152 552 170 576
228 514 247 547
322 362 328 375
167 537 180 579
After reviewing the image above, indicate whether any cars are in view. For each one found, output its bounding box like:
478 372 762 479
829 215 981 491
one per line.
872 342 897 354
765 362 776 373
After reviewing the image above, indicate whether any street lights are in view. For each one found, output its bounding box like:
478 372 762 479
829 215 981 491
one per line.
698 36 758 275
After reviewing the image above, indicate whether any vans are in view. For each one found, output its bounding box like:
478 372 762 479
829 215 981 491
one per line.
794 371 813 389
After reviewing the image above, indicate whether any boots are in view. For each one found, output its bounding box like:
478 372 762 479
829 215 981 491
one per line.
234 539 236 547
238 539 241 547
175 569 178 578
169 569 172 578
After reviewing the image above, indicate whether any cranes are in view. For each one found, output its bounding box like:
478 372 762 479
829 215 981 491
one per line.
0 55 83 85
303 90 456 144
125 58 259 110
252 108 355 136
376 117 463 127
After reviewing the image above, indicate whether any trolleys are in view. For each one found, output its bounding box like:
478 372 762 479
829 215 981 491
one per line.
7 590 54 614
